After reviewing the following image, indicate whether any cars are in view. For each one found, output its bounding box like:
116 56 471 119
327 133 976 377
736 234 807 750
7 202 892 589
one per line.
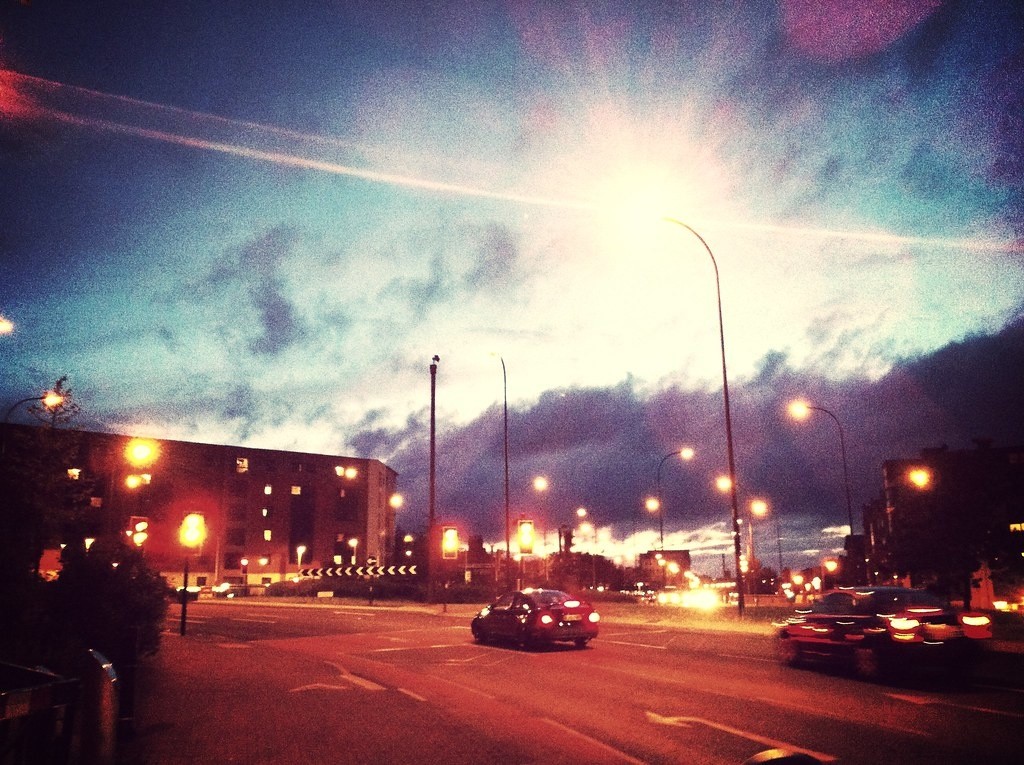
472 589 599 651
0 647 118 765
778 587 991 682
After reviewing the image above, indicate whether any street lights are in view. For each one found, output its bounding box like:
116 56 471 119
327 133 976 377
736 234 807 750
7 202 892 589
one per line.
579 509 596 590
651 207 746 617
297 546 305 566
791 404 854 535
2 394 63 452
349 539 356 565
538 479 547 586
750 501 783 569
489 350 511 592
719 478 754 558
658 449 691 586
242 559 247 597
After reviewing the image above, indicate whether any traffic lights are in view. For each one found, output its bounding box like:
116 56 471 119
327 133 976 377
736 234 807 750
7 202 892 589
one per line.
442 526 458 559
180 511 204 556
131 517 148 550
517 519 533 554
